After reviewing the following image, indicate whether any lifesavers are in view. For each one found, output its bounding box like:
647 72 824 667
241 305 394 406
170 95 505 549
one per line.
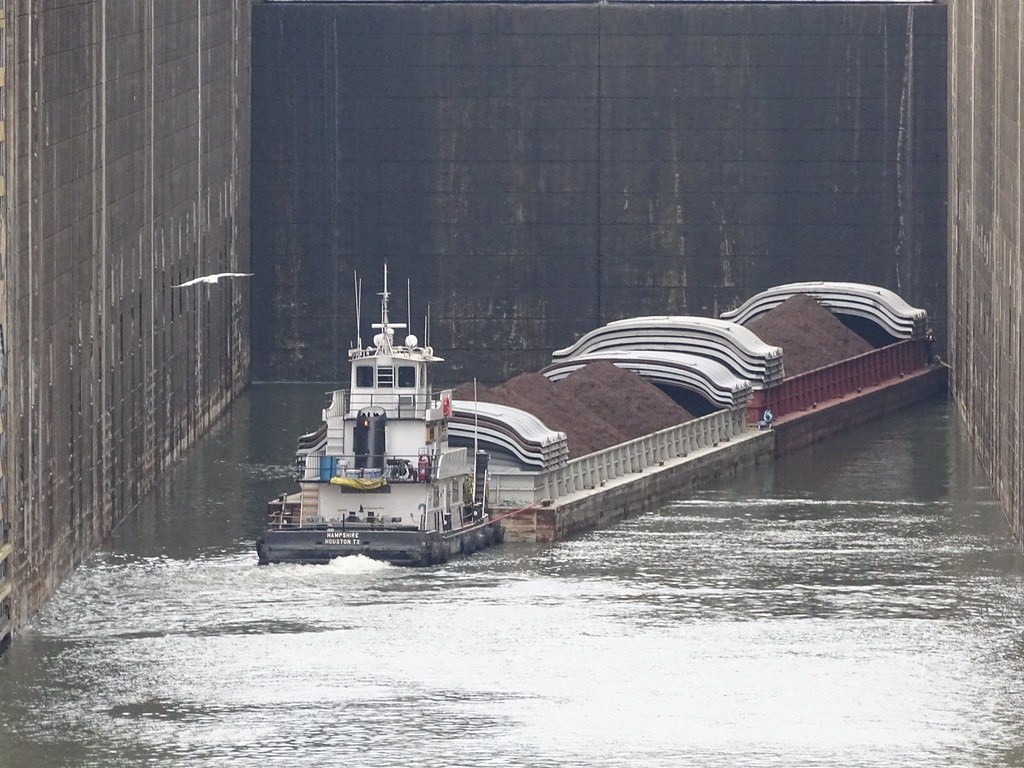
444 396 450 417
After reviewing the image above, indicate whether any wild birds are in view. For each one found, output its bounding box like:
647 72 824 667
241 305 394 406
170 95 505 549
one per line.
170 271 255 288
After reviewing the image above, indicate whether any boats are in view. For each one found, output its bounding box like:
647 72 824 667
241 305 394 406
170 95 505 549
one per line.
256 268 505 561
